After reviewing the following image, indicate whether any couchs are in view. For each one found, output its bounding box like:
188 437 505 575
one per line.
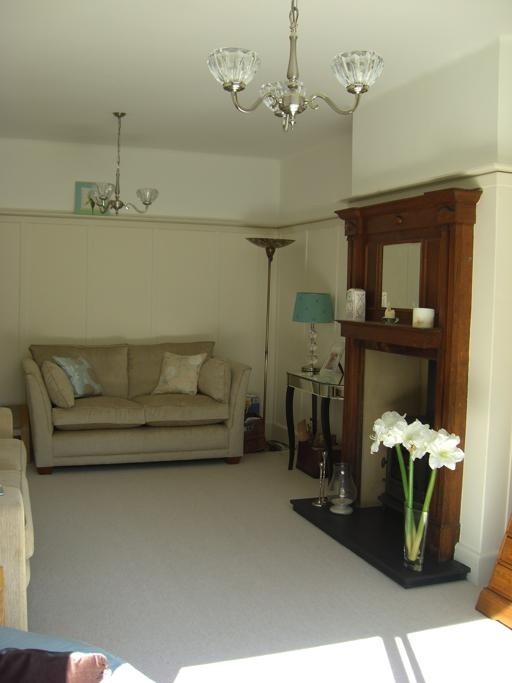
21 338 253 475
0 404 37 632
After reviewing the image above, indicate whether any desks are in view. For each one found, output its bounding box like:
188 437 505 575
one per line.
285 369 345 485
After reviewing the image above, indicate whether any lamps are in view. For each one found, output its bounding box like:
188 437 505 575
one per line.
247 236 296 452
293 290 334 370
207 0 386 130
84 107 159 216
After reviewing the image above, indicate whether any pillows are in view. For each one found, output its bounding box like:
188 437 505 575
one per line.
41 359 75 408
152 350 208 396
197 356 233 402
50 352 104 398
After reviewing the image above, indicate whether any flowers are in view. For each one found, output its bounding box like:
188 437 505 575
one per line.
367 408 466 562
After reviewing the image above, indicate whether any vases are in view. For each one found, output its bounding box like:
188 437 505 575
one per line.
402 498 439 570
328 462 360 513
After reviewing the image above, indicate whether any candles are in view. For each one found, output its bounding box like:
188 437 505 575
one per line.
384 307 394 316
